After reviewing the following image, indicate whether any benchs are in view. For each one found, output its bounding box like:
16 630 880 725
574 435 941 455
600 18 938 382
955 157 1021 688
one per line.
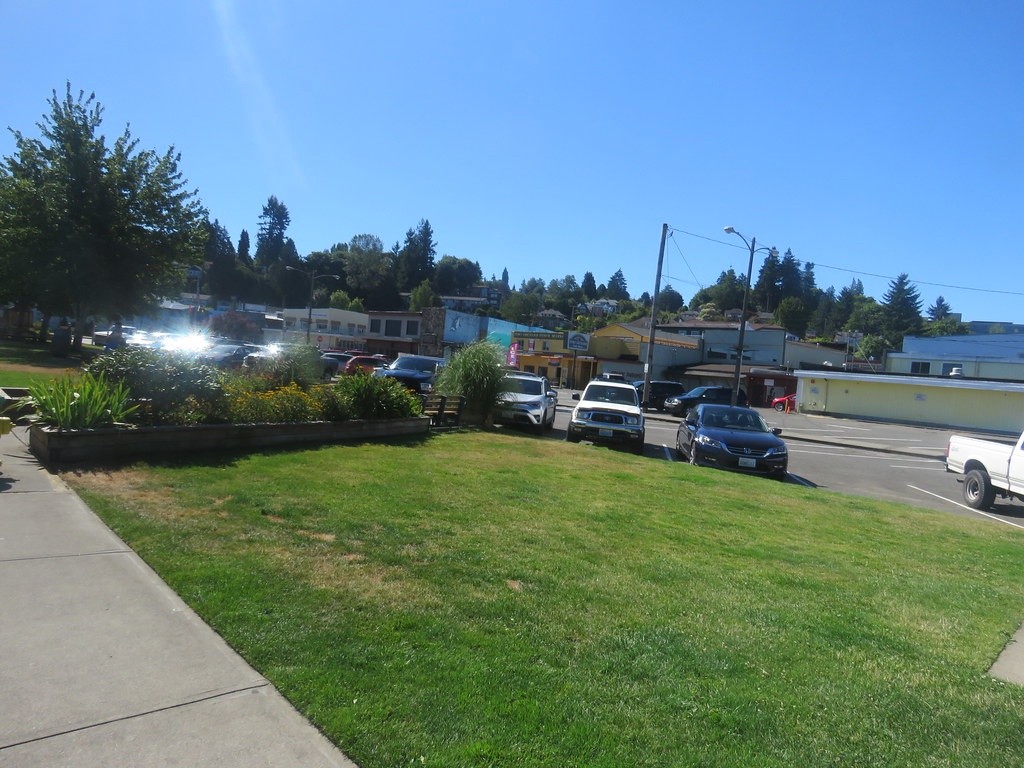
420 393 466 426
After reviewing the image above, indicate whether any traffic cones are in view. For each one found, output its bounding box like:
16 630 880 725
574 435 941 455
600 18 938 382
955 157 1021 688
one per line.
786 406 791 414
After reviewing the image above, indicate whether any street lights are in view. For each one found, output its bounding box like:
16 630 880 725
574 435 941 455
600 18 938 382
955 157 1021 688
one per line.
284 265 340 346
722 224 779 408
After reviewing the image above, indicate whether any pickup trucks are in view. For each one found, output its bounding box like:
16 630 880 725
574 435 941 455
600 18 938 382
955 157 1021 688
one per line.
944 427 1024 511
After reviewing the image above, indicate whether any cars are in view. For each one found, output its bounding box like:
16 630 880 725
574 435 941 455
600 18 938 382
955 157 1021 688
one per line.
771 392 796 412
674 404 789 481
91 324 523 403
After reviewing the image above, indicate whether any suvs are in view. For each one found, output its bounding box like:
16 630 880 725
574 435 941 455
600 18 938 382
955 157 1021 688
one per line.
664 385 750 419
490 373 558 434
608 381 686 411
567 377 647 454
